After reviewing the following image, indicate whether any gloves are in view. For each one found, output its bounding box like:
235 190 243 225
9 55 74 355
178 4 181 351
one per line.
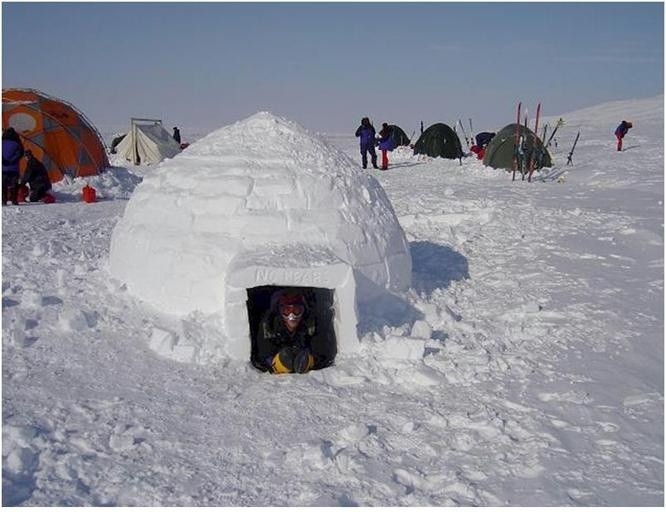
271 347 295 373
293 348 316 373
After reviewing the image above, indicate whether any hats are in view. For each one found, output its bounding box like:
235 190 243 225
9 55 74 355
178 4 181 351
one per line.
22 148 31 157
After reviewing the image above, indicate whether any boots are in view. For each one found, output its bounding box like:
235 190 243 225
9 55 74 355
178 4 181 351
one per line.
379 164 388 170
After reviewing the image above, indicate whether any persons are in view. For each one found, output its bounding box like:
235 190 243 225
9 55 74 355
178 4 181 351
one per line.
2 127 24 205
18 149 56 203
474 131 496 147
171 126 180 146
376 123 390 170
350 117 380 170
249 288 337 374
613 121 632 152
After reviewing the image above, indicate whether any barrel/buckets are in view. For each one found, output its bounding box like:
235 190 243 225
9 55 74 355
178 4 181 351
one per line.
82 186 96 203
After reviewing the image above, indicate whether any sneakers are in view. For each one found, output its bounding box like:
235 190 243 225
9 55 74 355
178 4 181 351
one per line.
362 165 379 169
2 199 20 205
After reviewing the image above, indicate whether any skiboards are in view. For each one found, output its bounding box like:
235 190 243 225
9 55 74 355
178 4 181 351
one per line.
513 102 581 183
457 117 475 148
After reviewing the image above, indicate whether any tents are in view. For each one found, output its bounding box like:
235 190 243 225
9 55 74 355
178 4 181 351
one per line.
2 85 110 184
112 116 182 165
388 125 410 149
412 121 466 160
483 124 555 174
